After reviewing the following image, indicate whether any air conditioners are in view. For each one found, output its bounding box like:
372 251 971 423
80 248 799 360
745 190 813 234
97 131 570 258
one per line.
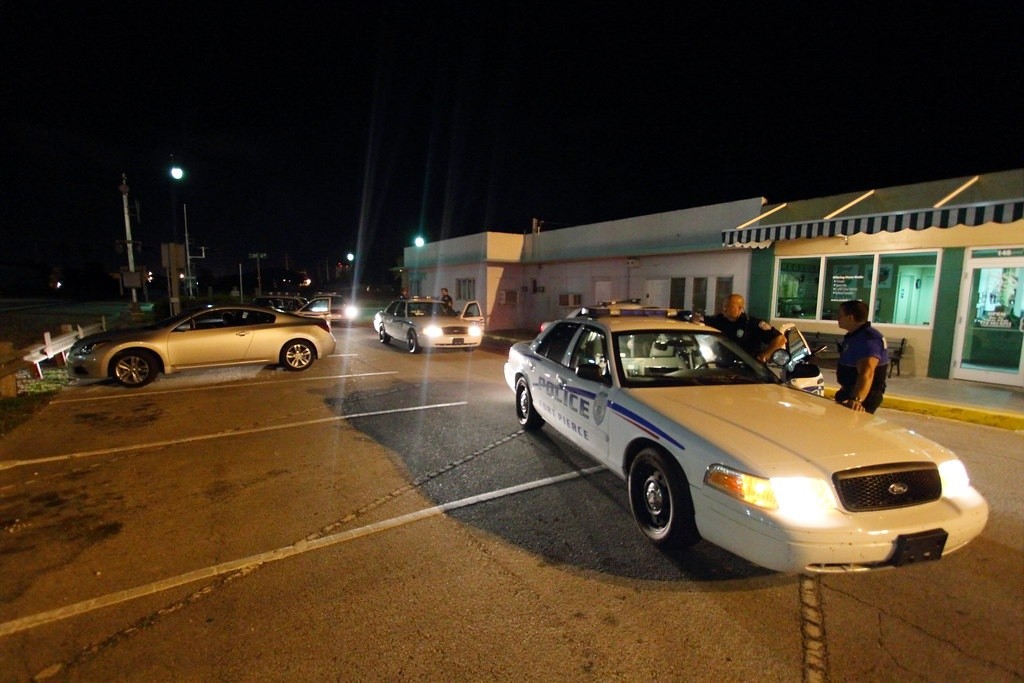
499 291 520 304
557 293 577 308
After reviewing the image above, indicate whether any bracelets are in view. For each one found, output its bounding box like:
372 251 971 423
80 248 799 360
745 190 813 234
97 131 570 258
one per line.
850 396 862 402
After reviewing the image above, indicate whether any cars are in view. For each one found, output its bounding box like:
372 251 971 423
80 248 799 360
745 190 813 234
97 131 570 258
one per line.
503 304 991 578
541 296 827 398
373 294 485 354
66 304 339 388
248 289 359 322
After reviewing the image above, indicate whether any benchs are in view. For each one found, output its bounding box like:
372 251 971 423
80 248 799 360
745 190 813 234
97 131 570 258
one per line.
800 331 906 378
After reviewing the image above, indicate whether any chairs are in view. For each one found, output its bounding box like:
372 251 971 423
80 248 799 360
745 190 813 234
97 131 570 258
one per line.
638 341 687 375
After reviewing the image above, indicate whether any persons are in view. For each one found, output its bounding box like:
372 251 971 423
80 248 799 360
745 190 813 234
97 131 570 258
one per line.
834 301 891 415
440 288 453 307
690 294 787 374
401 286 409 298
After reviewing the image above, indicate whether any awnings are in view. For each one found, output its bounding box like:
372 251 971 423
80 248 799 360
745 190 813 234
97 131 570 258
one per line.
721 176 1024 249
